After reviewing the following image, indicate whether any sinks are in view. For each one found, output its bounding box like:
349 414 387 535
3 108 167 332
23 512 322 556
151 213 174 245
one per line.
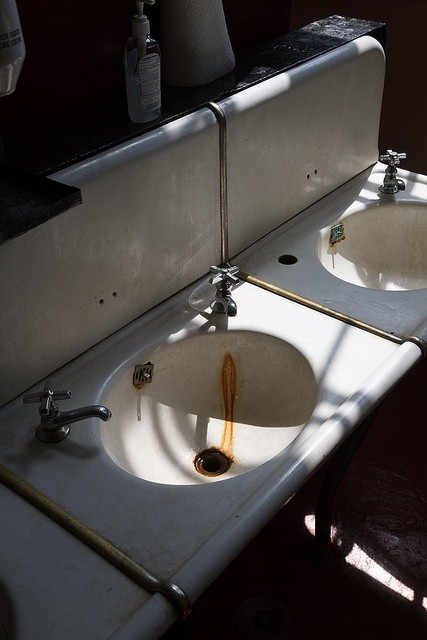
316 202 427 292
1 576 18 640
96 331 318 487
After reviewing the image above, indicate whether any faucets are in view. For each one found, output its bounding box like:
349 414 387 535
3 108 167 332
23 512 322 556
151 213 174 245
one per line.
379 150 407 194
208 264 240 317
22 379 112 444
